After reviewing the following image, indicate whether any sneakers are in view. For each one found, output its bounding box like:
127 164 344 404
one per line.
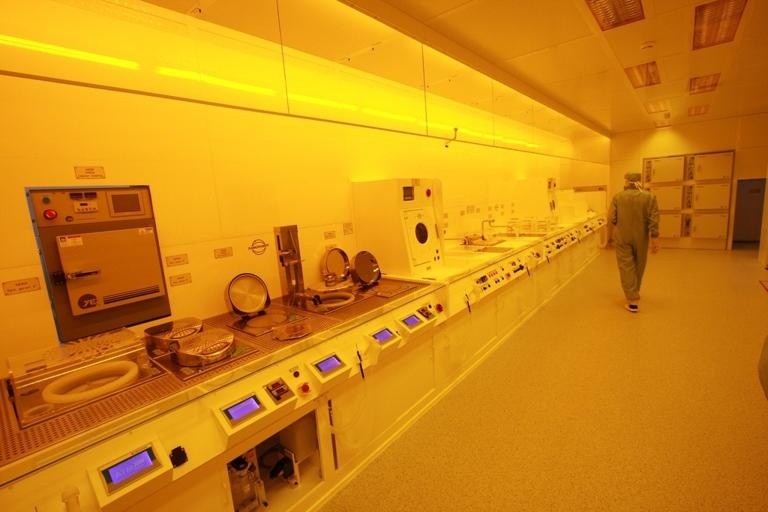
624 303 638 312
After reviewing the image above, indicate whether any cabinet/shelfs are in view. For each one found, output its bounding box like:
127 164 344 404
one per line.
641 149 736 252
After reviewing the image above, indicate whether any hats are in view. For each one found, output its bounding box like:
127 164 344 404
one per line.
623 172 642 182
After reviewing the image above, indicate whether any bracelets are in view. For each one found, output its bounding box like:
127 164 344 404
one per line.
607 238 613 244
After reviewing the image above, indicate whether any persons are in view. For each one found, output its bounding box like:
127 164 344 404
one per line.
605 171 661 313
